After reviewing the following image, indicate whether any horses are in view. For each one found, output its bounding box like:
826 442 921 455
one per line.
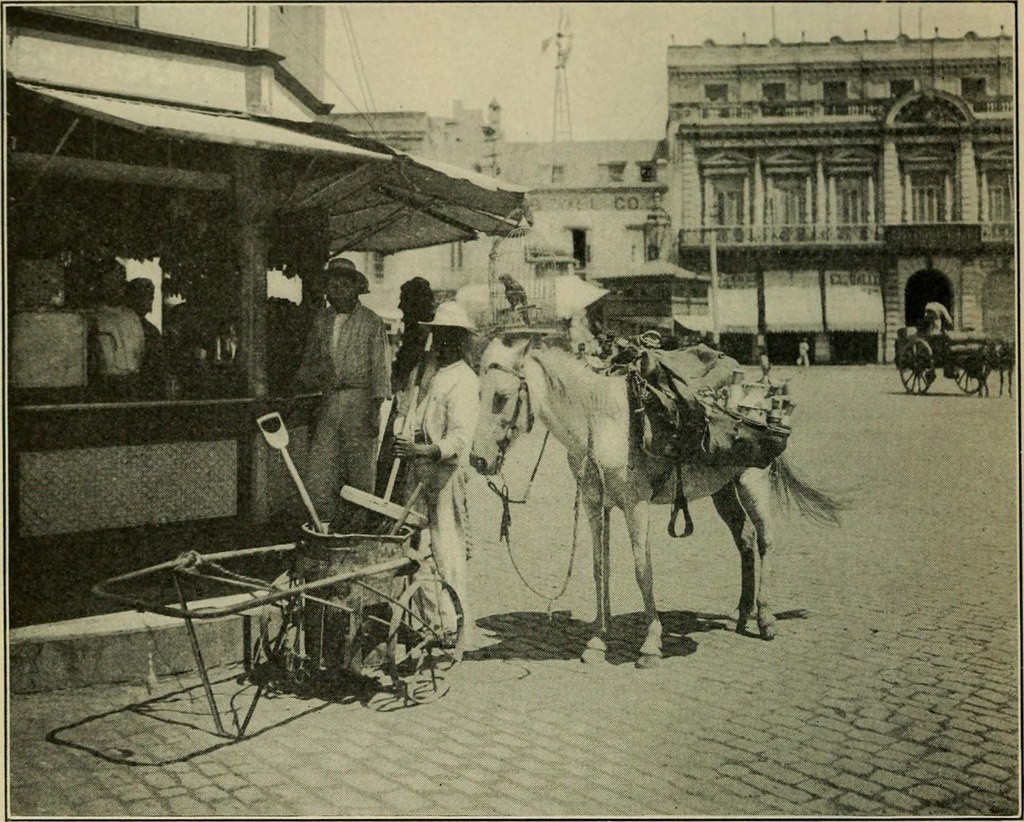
468 330 856 669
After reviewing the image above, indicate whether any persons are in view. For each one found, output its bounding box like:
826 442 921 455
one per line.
796 336 811 366
119 275 172 388
82 256 144 388
387 300 480 656
297 256 391 537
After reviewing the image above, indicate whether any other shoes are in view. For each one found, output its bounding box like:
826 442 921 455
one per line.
437 627 459 647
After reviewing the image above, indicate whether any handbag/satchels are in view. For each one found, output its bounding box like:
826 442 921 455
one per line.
633 342 792 469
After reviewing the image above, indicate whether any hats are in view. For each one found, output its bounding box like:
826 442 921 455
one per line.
313 258 369 295
418 303 477 336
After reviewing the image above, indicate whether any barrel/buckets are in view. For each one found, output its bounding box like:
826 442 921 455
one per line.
301 522 413 680
